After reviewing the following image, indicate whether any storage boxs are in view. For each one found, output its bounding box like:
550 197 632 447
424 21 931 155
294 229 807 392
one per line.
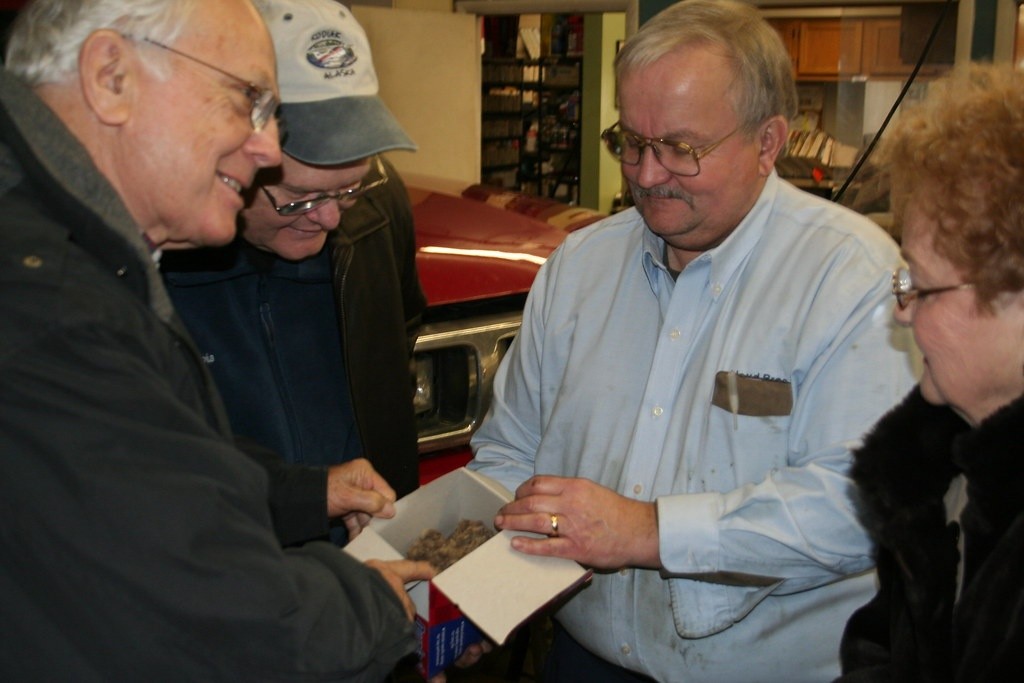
343 468 594 680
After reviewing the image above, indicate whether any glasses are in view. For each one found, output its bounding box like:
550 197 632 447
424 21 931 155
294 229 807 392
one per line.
893 267 1024 311
601 115 770 177
257 153 388 217
123 34 281 132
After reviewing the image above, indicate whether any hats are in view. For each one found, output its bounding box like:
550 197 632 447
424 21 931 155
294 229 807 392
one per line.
252 0 417 165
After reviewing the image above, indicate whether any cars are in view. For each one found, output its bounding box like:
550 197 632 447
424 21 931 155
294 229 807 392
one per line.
390 167 611 488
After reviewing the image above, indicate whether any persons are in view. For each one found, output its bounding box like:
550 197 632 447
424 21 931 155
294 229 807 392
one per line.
466 1 929 683
152 0 429 548
834 80 1024 683
0 0 438 683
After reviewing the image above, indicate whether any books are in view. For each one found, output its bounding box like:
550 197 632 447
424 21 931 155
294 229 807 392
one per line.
482 64 579 199
774 129 860 179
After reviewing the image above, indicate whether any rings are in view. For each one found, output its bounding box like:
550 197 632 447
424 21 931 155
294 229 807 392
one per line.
549 513 559 538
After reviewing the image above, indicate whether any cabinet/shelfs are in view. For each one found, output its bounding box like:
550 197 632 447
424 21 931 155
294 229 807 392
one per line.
480 53 584 202
764 18 959 80
